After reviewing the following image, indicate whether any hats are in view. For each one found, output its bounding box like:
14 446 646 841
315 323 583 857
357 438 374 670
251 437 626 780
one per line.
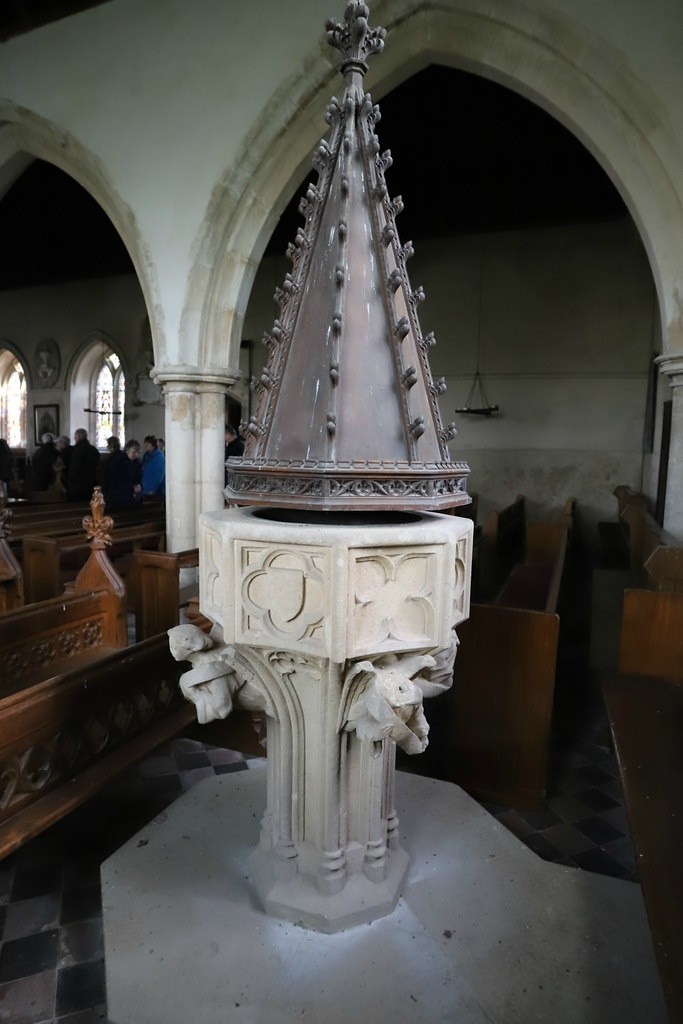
53 436 70 443
42 432 54 443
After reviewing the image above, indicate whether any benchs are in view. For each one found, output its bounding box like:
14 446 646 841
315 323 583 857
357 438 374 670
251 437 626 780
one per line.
0 483 683 861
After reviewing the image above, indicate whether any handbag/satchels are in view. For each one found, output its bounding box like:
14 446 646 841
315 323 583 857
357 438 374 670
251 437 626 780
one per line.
133 493 143 503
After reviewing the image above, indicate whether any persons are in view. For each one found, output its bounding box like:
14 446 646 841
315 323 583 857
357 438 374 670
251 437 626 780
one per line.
0 439 15 482
225 393 245 487
31 429 165 506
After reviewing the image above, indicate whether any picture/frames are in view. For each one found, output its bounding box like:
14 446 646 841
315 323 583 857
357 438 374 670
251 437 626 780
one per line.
33 404 61 446
